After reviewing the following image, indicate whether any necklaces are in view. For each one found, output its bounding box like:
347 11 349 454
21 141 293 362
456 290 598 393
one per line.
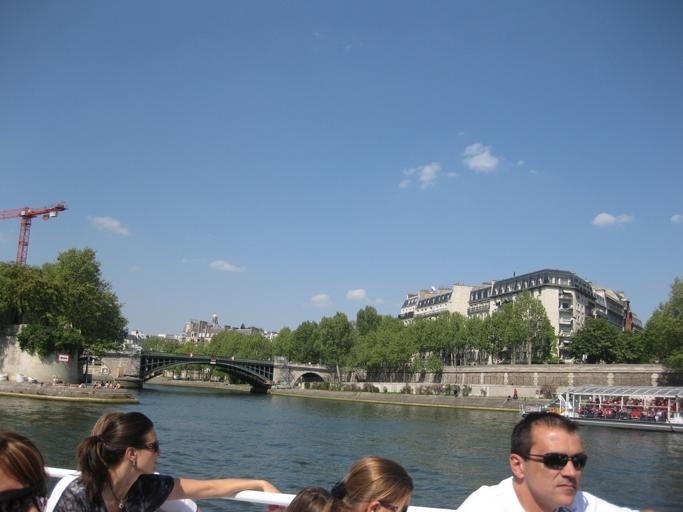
109 482 129 511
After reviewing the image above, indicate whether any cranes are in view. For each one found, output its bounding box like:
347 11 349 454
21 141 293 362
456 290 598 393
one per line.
0 203 66 265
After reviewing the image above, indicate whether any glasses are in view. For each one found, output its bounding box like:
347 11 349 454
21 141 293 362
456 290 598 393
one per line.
382 502 407 512
519 451 589 471
130 440 160 452
0 486 34 511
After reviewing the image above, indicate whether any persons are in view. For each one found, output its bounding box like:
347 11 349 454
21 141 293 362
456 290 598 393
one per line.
320 456 415 512
286 485 334 511
1 426 51 511
571 399 680 422
53 411 283 512
455 411 659 512
79 380 122 394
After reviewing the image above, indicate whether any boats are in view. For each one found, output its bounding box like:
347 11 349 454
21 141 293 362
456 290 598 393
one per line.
521 387 683 433
